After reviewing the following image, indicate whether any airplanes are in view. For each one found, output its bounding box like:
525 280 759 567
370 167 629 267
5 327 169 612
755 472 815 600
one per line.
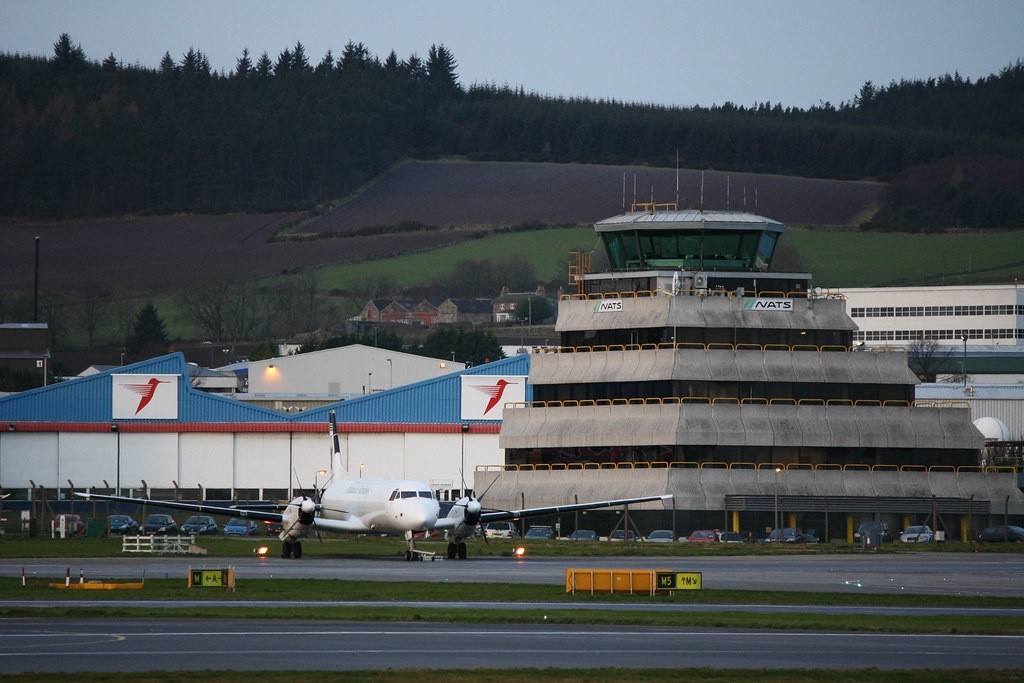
72 410 673 561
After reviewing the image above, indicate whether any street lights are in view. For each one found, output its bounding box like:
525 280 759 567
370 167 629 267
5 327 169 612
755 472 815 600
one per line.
775 468 780 529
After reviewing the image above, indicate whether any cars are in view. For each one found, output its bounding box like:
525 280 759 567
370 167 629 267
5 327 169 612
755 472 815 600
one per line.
52 514 84 535
978 526 1024 544
686 530 719 542
223 517 258 537
854 522 893 543
108 514 138 535
524 525 555 539
900 526 933 543
566 529 600 541
483 523 521 539
716 532 743 543
766 528 802 543
647 530 680 543
608 530 639 541
180 516 218 535
267 522 284 537
139 514 178 537
